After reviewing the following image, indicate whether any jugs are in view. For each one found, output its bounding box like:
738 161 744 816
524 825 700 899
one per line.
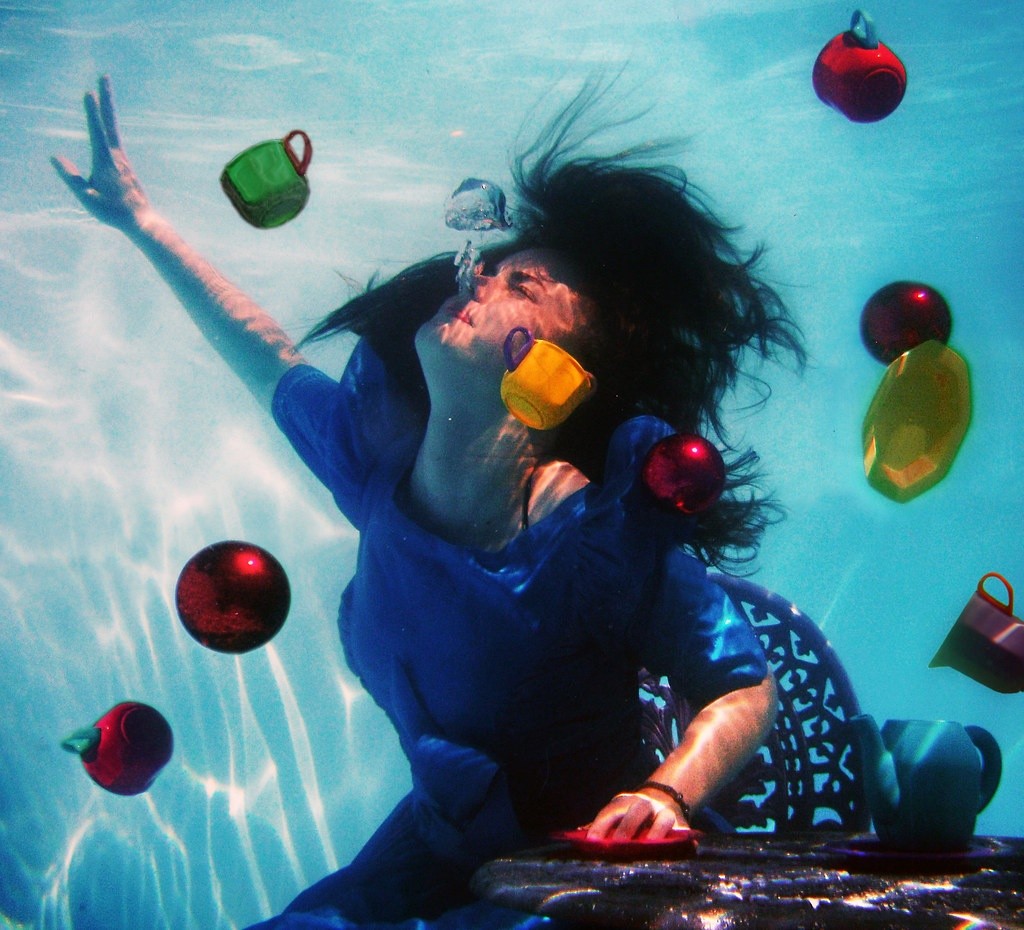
848 715 1004 853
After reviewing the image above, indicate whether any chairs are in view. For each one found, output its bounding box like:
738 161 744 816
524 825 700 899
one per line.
638 573 903 838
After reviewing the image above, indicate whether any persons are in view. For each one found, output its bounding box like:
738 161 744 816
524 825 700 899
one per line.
48 74 809 930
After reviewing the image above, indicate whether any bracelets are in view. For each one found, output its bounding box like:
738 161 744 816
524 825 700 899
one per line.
639 780 690 823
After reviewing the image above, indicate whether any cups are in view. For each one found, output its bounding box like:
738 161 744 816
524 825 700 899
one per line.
933 573 1024 694
224 129 313 228
815 11 904 122
505 327 596 428
63 702 174 793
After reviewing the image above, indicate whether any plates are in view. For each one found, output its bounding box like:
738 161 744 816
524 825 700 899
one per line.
818 835 1014 866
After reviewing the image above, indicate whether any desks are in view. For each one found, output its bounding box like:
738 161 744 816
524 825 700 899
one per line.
474 833 1024 930
